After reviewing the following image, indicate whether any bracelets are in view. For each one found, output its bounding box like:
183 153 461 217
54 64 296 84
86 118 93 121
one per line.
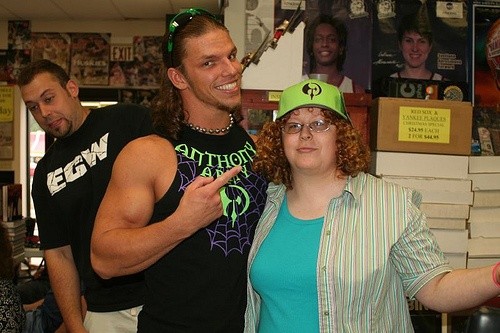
492 263 500 288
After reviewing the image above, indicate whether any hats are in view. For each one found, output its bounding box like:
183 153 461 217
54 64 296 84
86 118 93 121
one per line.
276 78 351 123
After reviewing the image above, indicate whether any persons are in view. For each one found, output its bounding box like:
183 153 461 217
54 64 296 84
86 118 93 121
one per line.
387 13 450 80
23 285 87 333
284 14 366 94
90 7 271 333
242 79 500 333
0 222 26 333
17 60 165 333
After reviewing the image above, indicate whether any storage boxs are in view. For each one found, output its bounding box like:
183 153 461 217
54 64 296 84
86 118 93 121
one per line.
370 97 473 156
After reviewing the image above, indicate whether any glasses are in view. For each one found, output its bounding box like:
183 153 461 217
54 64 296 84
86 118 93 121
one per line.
168 7 222 69
277 119 338 133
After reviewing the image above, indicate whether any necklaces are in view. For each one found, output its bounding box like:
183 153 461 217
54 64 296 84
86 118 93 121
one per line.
183 113 234 134
